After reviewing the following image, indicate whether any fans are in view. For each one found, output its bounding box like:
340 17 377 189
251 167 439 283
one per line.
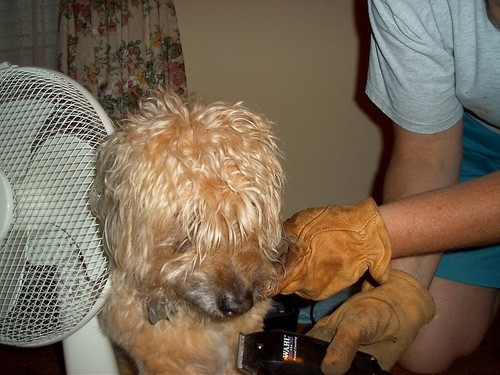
0 62 121 375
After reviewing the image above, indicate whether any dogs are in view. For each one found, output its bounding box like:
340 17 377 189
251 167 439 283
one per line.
88 87 291 375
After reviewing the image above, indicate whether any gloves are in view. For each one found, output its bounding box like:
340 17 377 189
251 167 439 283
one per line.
303 267 436 375
260 196 390 300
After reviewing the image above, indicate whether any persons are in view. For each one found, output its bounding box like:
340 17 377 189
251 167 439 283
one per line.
266 0 500 375
